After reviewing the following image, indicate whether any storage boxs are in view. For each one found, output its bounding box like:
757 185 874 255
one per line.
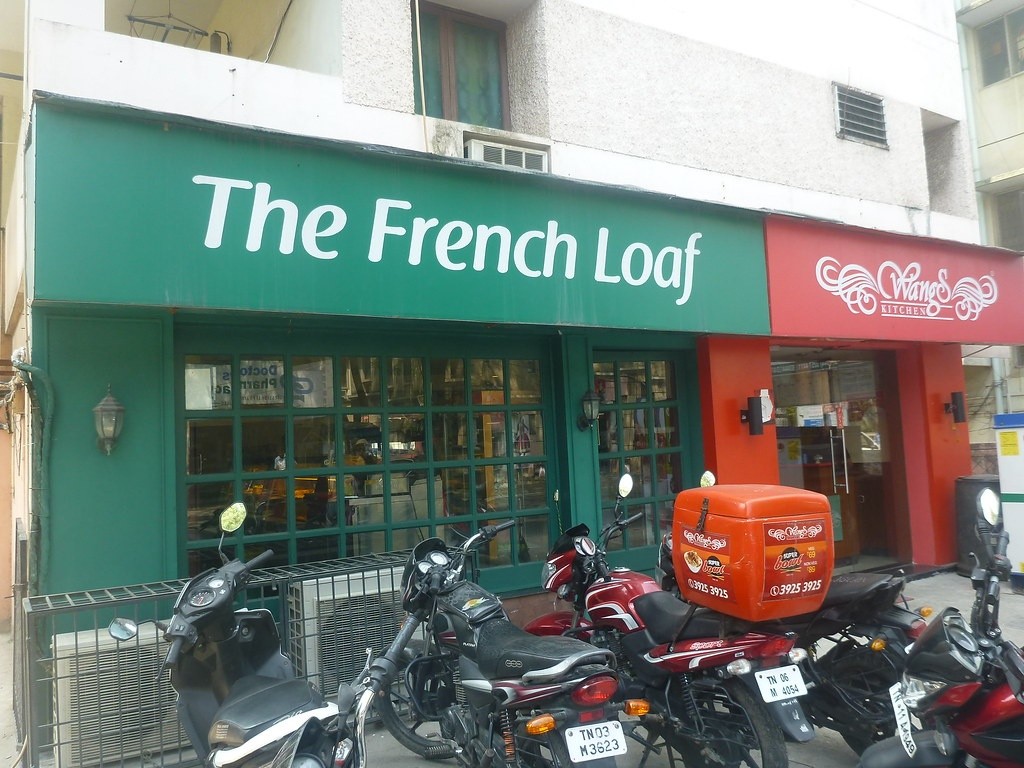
790 404 824 427
672 483 835 621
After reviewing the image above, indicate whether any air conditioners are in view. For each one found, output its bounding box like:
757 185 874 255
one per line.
50 618 194 768
463 140 548 171
287 564 435 698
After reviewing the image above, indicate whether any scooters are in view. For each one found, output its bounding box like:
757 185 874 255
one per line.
109 502 359 768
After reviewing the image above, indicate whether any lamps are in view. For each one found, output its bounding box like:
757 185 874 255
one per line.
740 397 764 435
945 392 965 423
92 384 126 457
210 30 231 53
576 387 601 432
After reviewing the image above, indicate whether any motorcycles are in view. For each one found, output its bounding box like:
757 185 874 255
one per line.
521 473 834 768
855 487 1024 768
444 482 531 567
198 489 363 573
654 471 931 759
375 519 649 768
